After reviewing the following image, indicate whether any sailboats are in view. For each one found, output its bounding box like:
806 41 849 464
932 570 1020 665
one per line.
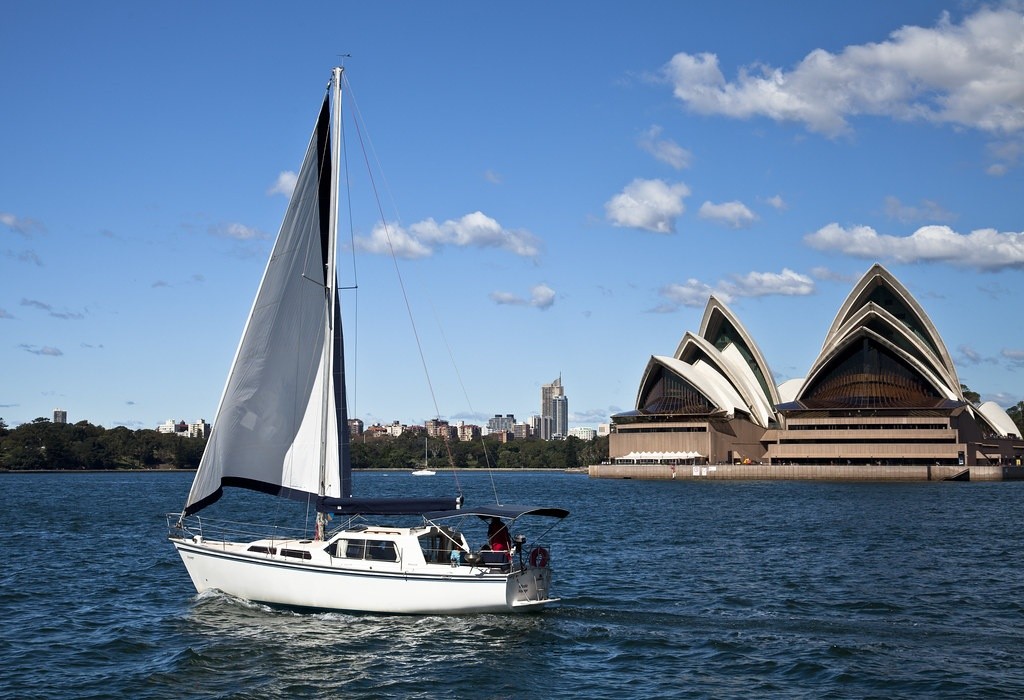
411 437 436 476
162 67 577 618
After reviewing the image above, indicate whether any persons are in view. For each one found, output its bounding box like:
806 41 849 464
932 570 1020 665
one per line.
451 544 468 566
487 518 512 563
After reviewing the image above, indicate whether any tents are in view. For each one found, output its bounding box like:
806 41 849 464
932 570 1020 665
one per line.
625 451 704 465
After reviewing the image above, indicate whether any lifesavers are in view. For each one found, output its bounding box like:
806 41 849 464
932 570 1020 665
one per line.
529 548 549 568
